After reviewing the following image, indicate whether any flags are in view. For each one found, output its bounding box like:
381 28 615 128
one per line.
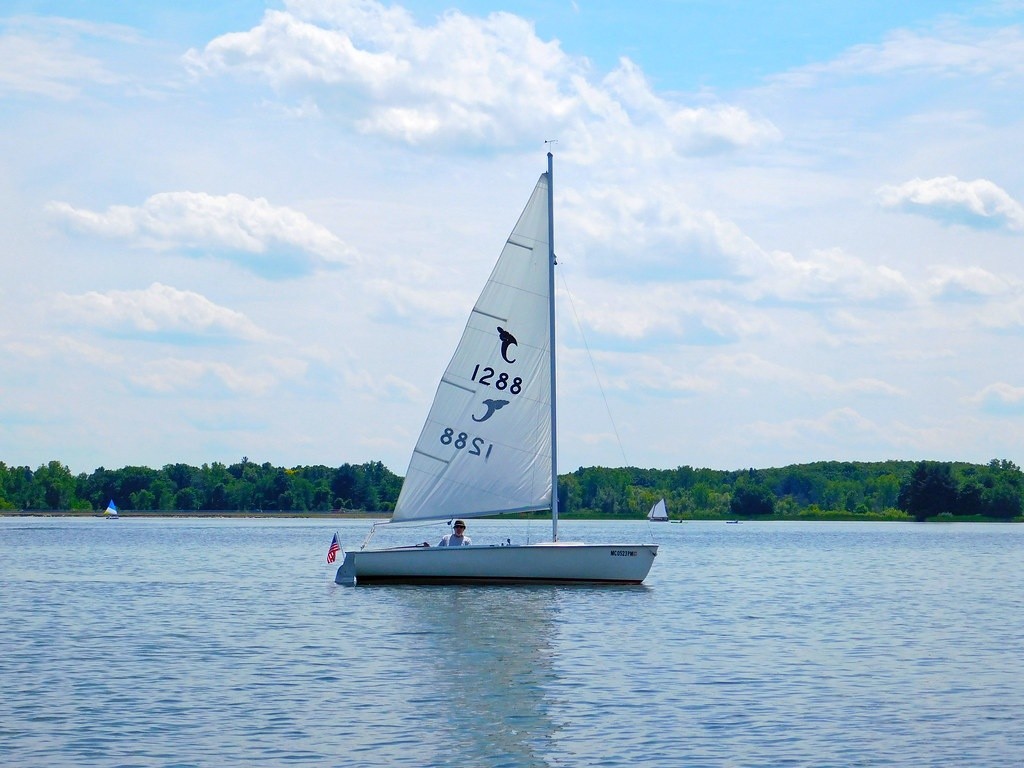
327 534 339 563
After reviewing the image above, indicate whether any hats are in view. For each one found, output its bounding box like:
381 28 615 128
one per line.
453 520 466 529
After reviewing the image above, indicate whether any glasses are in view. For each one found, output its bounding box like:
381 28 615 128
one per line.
454 526 463 529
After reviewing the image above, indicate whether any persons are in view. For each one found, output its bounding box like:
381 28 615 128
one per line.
437 520 472 546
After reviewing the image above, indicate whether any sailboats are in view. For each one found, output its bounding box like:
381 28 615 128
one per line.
647 498 668 521
102 498 119 519
334 139 661 589
